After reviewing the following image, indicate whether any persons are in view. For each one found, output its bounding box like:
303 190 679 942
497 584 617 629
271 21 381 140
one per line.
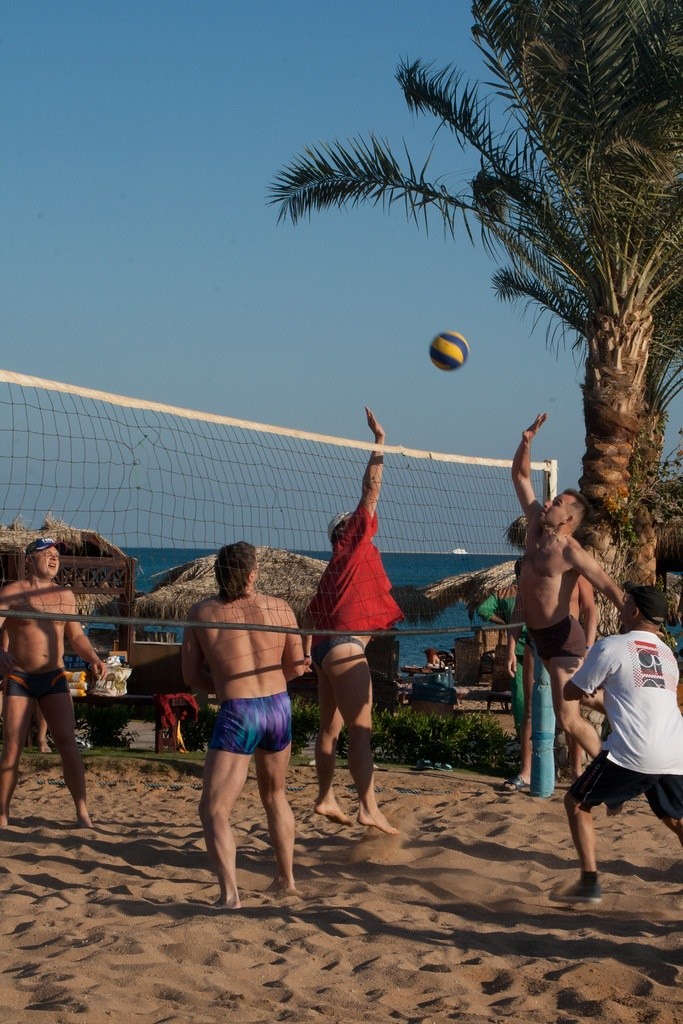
477 557 525 730
181 541 305 909
0 538 108 829
550 581 683 902
513 413 625 758
424 649 444 669
501 516 597 792
300 407 399 835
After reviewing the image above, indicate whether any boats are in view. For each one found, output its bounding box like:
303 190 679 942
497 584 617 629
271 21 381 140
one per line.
453 549 467 554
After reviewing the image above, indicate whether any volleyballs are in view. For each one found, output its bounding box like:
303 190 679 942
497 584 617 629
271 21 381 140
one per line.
429 331 471 372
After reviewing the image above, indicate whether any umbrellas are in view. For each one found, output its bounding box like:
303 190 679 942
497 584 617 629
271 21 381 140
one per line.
133 546 329 620
391 560 683 630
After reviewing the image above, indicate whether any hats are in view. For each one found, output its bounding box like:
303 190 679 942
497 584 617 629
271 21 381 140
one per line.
24 538 67 557
328 511 350 541
623 580 667 625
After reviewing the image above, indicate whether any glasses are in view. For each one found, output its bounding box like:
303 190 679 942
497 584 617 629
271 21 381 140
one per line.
622 594 632 602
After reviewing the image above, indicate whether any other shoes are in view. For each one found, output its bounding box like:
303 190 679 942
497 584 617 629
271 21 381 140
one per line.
550 872 603 904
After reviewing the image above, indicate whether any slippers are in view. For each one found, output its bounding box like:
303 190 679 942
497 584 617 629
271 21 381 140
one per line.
503 775 530 791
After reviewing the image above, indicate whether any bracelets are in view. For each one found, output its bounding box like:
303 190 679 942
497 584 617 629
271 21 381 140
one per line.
304 653 310 658
586 646 591 650
